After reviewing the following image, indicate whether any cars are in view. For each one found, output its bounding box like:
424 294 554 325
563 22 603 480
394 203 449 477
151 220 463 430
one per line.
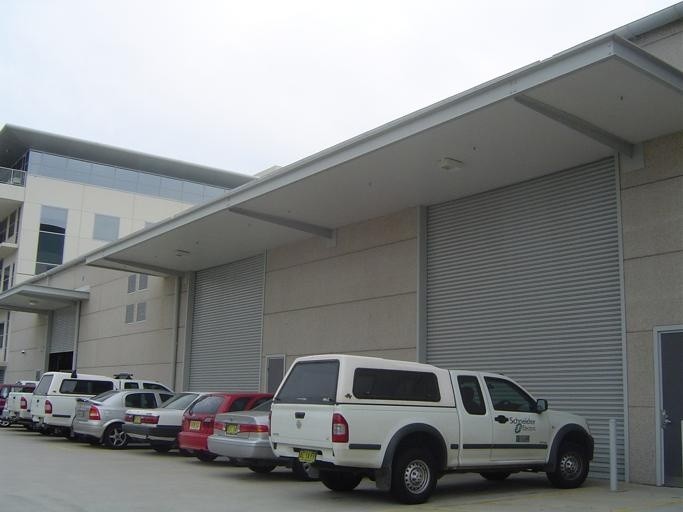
73 388 322 481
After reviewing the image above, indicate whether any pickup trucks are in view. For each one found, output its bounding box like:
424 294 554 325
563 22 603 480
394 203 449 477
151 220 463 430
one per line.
269 354 594 504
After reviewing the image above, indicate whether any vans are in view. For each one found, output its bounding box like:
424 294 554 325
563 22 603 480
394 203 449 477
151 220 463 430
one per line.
1 370 175 433
